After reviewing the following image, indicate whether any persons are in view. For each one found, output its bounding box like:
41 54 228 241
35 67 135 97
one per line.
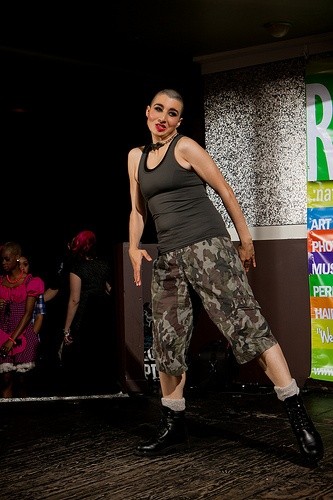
126 87 326 464
0 229 113 399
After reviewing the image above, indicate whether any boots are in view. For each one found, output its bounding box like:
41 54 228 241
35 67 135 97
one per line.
134 397 192 456
274 379 325 461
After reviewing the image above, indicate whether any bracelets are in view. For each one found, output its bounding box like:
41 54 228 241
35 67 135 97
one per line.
58 328 72 360
7 336 17 345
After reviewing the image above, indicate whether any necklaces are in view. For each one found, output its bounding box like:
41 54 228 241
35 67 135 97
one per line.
4 270 25 284
146 132 179 152
